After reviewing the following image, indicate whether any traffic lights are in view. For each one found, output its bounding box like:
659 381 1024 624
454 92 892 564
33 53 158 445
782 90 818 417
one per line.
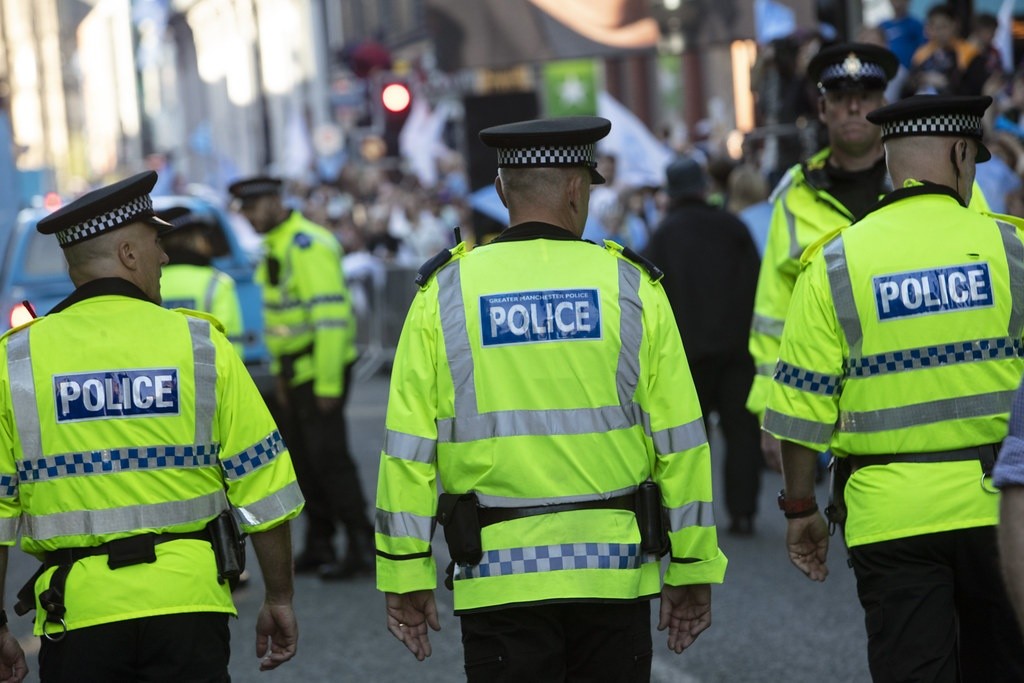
376 77 413 156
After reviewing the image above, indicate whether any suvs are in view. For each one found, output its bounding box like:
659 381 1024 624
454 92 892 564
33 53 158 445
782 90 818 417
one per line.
0 194 274 418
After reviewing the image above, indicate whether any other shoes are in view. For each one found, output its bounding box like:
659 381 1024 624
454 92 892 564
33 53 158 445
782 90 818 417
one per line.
320 545 377 578
293 546 336 575
728 515 752 534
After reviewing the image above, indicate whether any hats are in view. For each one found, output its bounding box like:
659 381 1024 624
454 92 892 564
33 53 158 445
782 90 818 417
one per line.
155 205 215 236
479 116 612 184
866 95 993 163
228 178 282 214
807 42 900 91
37 170 174 249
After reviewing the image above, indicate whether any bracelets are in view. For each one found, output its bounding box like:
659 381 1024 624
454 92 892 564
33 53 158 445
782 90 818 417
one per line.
776 489 817 511
783 503 818 516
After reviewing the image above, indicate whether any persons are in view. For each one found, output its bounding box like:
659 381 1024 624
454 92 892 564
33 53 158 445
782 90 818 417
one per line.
376 115 729 683
762 95 1024 683
993 375 1024 625
1 169 306 681
142 0 1024 580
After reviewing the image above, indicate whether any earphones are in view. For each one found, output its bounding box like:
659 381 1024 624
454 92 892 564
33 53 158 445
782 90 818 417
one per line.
962 151 965 161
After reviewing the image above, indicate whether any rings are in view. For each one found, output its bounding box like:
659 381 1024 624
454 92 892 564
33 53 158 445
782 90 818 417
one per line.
798 554 803 559
399 623 406 626
